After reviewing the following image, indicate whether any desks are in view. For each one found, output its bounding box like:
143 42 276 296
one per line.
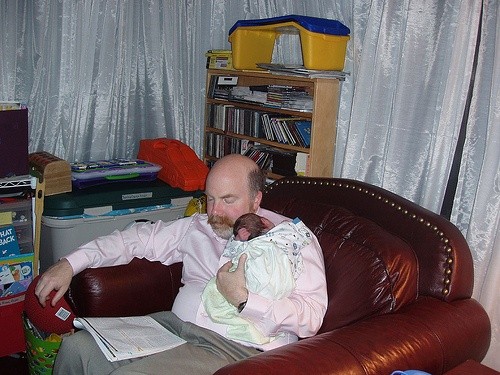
440 359 500 375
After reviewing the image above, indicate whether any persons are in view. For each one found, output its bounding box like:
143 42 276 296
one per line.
233 213 313 298
34 154 328 375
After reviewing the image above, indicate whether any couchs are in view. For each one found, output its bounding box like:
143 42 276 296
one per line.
24 176 490 375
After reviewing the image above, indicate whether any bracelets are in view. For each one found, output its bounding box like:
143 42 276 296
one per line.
237 299 248 313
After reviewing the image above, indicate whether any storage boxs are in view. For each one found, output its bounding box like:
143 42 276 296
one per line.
22 313 73 375
29 151 72 195
39 207 178 278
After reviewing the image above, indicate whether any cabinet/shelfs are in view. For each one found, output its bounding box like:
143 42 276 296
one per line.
203 68 340 178
0 171 45 307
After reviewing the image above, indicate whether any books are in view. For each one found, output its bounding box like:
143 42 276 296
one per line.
206 62 351 174
0 212 35 301
